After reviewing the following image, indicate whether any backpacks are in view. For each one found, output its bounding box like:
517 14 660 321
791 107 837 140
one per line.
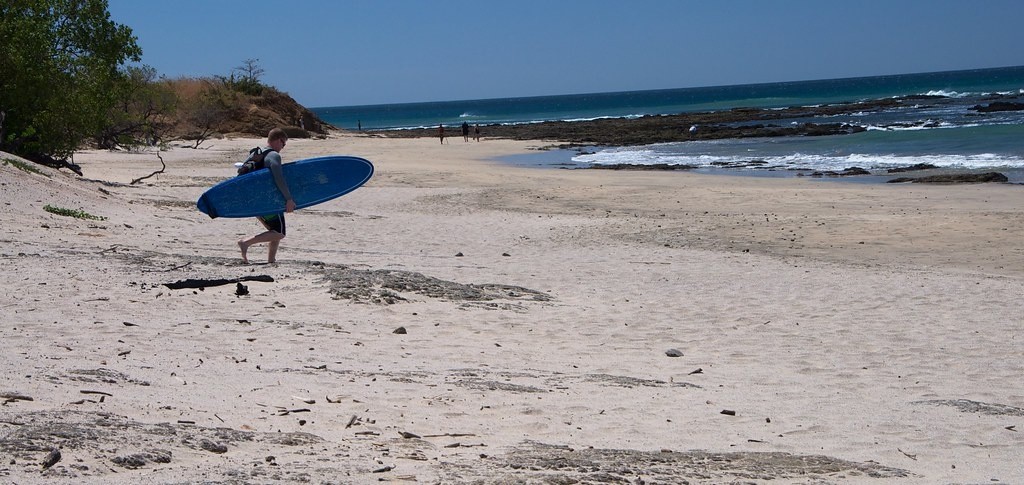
237 146 279 176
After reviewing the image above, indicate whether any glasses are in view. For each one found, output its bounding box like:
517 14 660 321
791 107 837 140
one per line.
280 138 286 148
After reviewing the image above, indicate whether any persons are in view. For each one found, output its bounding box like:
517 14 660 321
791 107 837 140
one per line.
463 122 469 142
238 129 297 264
358 120 361 130
473 123 480 142
439 124 444 144
